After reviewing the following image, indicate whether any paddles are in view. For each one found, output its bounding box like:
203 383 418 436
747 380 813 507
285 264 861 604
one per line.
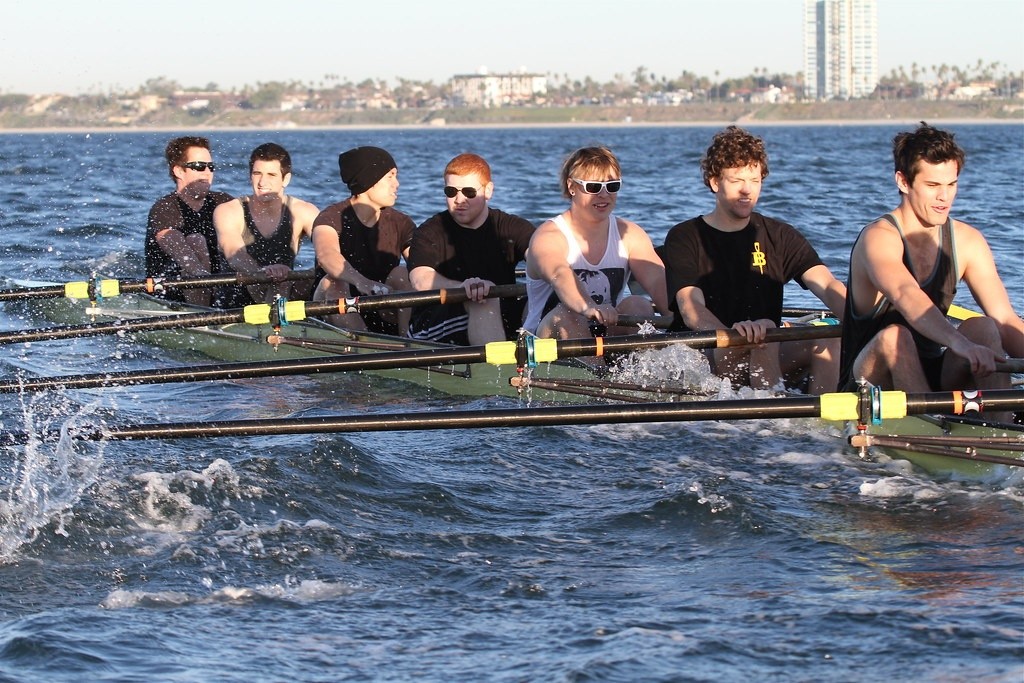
0 388 1023 446
0 321 843 392
0 270 319 303
0 280 529 345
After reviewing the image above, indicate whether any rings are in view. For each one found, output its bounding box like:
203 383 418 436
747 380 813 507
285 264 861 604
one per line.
470 283 478 289
478 282 484 288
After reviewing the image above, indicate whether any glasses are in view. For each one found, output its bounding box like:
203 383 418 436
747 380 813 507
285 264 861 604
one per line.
178 161 215 172
571 177 623 194
443 184 484 199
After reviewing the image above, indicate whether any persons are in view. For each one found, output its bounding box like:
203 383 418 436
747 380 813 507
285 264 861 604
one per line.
143 136 236 306
836 122 1024 425
310 146 418 338
664 125 847 395
406 154 537 345
520 145 674 365
211 141 321 308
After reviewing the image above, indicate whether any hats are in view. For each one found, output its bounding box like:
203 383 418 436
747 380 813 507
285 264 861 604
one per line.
338 146 398 195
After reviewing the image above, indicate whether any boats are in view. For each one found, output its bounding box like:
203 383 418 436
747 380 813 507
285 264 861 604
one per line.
3 276 1024 497
